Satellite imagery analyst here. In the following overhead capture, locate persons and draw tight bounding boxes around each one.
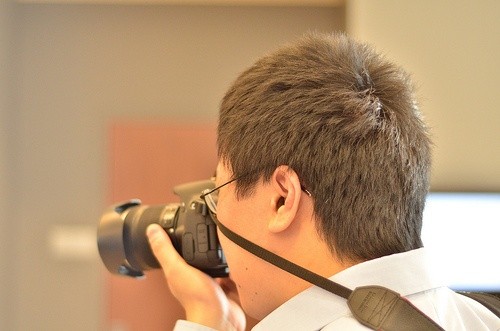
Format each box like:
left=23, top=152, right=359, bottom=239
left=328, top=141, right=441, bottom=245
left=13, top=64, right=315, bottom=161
left=146, top=31, right=500, bottom=330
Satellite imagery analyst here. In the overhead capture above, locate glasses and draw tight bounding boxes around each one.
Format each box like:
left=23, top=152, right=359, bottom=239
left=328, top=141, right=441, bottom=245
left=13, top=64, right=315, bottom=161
left=200, top=164, right=312, bottom=217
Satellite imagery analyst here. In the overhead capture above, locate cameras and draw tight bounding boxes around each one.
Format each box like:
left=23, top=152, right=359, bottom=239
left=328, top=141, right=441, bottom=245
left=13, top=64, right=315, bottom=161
left=96, top=179, right=230, bottom=279
